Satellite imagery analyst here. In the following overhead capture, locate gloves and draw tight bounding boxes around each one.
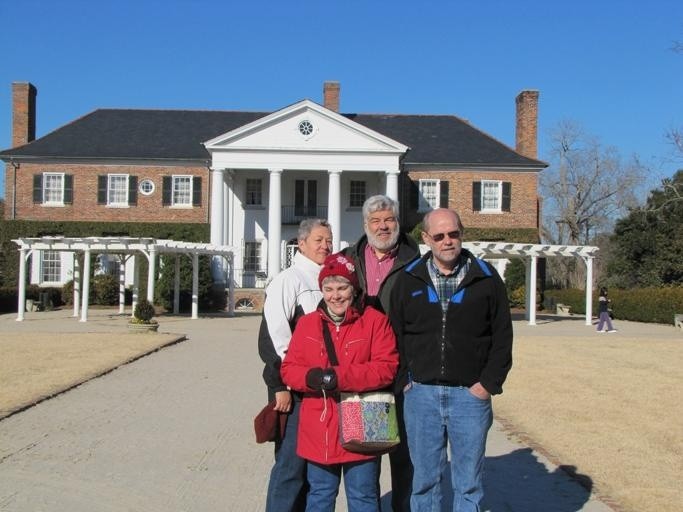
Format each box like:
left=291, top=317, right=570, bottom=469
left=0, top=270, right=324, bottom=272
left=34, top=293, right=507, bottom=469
left=306, top=368, right=337, bottom=390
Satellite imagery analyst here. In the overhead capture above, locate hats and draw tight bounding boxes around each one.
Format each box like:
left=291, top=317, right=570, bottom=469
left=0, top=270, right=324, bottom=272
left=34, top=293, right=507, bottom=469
left=318, top=253, right=360, bottom=292
left=254, top=400, right=288, bottom=443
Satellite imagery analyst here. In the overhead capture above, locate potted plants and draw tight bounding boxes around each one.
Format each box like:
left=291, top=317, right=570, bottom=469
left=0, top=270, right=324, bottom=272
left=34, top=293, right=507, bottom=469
left=128, top=299, right=158, bottom=333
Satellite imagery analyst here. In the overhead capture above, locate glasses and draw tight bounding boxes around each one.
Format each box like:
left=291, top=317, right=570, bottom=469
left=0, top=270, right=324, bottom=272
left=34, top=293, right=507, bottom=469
left=427, top=231, right=460, bottom=241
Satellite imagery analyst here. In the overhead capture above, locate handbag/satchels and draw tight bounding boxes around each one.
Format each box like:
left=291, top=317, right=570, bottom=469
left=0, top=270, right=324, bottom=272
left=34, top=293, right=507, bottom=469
left=338, top=391, right=401, bottom=455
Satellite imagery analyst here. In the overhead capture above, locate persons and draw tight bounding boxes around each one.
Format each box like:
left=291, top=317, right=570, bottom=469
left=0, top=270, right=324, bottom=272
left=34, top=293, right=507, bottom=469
left=256, top=216, right=338, bottom=511
left=279, top=251, right=399, bottom=512
left=595, top=287, right=618, bottom=332
left=385, top=206, right=513, bottom=512
left=329, top=193, right=423, bottom=512
